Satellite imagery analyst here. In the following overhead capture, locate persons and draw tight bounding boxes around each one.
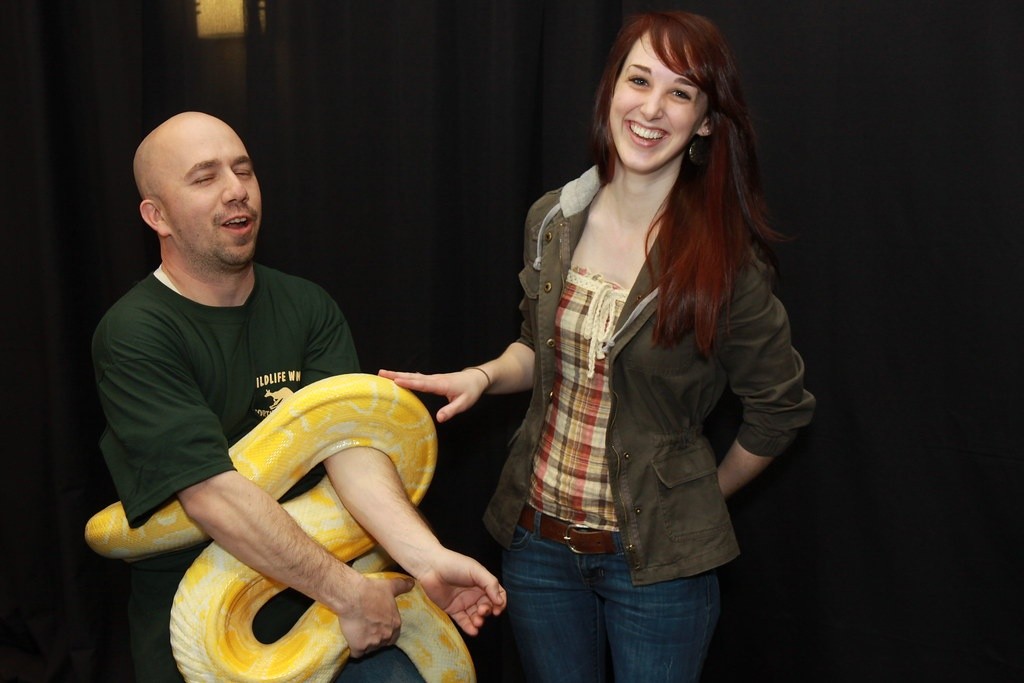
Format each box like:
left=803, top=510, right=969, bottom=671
left=377, top=11, right=816, bottom=683
left=91, top=111, right=507, bottom=683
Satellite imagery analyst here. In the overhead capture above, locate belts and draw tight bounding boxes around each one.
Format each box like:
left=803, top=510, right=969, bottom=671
left=517, top=505, right=617, bottom=554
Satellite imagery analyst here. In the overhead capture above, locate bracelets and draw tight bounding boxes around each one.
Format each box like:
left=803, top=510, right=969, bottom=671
left=464, top=367, right=491, bottom=385
